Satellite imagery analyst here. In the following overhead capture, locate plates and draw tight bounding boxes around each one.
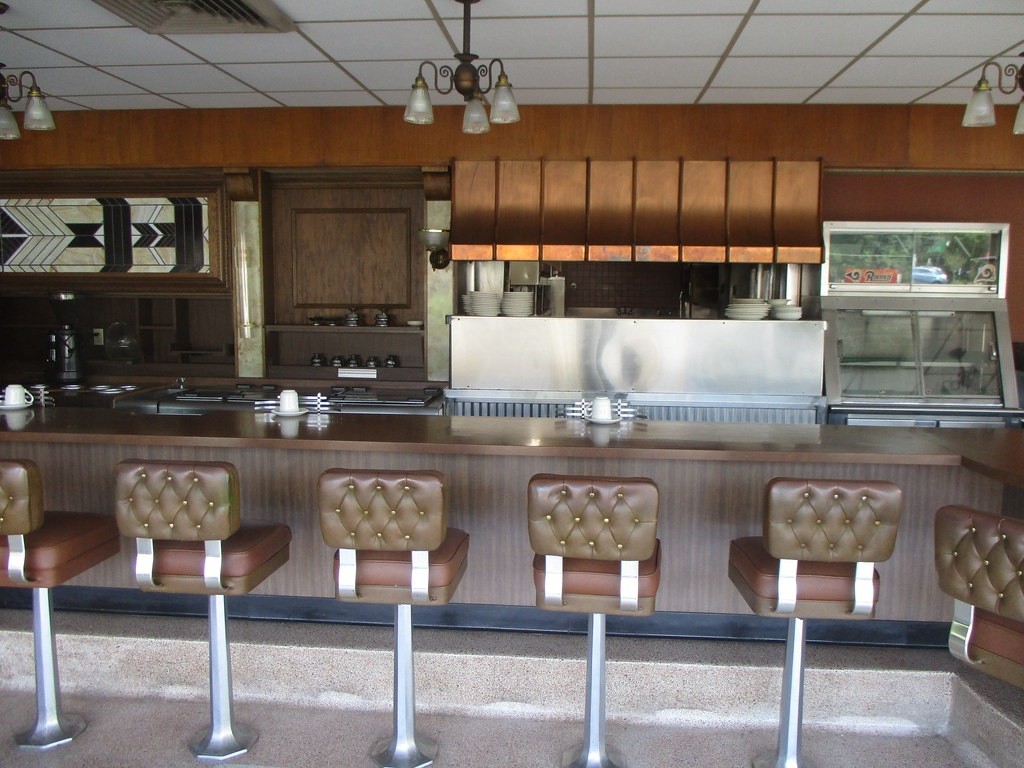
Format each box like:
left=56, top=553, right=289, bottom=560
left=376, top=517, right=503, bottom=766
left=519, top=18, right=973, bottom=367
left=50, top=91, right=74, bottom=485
left=502, top=292, right=534, bottom=316
left=271, top=408, right=308, bottom=417
left=725, top=298, right=771, bottom=320
left=586, top=416, right=622, bottom=424
left=0, top=401, right=33, bottom=410
left=407, top=321, right=423, bottom=326
left=462, top=291, right=501, bottom=316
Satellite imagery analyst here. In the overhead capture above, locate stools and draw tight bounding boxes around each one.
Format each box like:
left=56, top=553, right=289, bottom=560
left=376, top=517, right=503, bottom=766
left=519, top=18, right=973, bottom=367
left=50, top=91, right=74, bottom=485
left=112, top=457, right=292, bottom=762
left=728, top=477, right=905, bottom=768
left=0, top=457, right=122, bottom=751
left=314, top=469, right=470, bottom=768
left=528, top=472, right=660, bottom=768
left=934, top=500, right=1024, bottom=690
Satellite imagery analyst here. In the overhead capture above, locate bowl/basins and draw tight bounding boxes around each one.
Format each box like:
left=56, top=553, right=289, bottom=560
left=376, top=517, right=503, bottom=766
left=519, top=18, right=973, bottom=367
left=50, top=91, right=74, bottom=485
left=763, top=299, right=790, bottom=306
left=770, top=307, right=802, bottom=320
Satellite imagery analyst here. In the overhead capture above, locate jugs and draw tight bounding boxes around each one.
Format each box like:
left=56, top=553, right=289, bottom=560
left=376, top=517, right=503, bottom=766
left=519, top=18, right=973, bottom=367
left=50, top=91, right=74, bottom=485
left=58, top=324, right=82, bottom=384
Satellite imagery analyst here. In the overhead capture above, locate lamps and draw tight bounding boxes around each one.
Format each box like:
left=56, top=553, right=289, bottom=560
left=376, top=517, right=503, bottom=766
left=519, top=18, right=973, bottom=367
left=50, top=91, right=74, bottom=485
left=418, top=227, right=451, bottom=272
left=403, top=1, right=522, bottom=133
left=0, top=3, right=56, bottom=141
left=961, top=52, right=1024, bottom=142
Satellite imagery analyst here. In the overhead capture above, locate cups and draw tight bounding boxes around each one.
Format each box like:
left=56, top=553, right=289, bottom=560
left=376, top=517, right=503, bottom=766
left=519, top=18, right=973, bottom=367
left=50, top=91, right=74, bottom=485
left=280, top=390, right=298, bottom=412
left=5, top=385, right=34, bottom=405
left=586, top=397, right=612, bottom=420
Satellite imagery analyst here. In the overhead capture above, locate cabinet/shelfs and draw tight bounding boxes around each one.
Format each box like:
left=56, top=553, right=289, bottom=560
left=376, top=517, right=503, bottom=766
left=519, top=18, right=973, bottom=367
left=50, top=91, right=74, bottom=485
left=264, top=323, right=424, bottom=382
left=0, top=296, right=235, bottom=366
